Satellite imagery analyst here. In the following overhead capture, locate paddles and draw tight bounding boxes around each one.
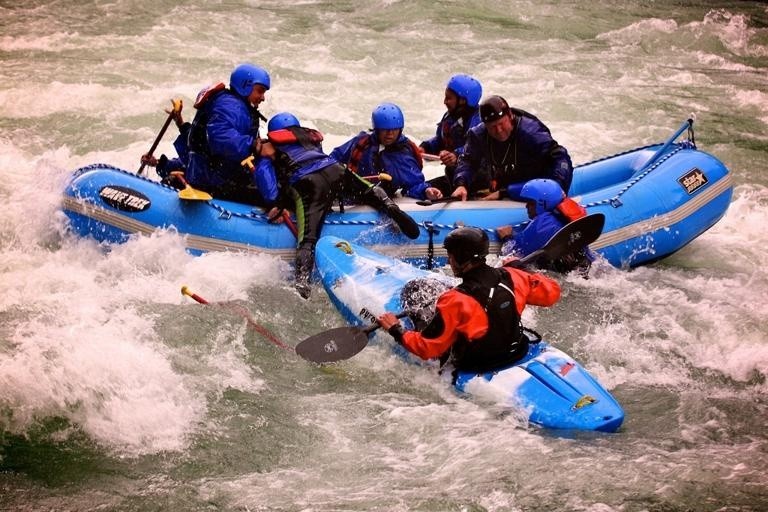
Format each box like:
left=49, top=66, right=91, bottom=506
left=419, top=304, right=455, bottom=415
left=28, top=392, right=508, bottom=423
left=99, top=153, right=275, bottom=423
left=171, top=170, right=212, bottom=200
left=296, top=213, right=608, bottom=366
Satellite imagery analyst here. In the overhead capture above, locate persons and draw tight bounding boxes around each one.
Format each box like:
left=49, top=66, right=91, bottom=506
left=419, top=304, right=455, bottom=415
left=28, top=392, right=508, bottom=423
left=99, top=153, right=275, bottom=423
left=418, top=74, right=482, bottom=198
left=141, top=99, right=191, bottom=191
left=183, top=63, right=270, bottom=205
left=449, top=95, right=574, bottom=198
left=482, top=179, right=596, bottom=280
left=376, top=227, right=560, bottom=372
left=328, top=103, right=443, bottom=201
left=267, top=112, right=420, bottom=300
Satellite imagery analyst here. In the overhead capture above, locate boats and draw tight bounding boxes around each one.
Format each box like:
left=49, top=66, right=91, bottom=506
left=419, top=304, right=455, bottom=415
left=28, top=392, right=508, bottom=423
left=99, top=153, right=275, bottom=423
left=314, top=235, right=625, bottom=434
left=62, top=117, right=734, bottom=275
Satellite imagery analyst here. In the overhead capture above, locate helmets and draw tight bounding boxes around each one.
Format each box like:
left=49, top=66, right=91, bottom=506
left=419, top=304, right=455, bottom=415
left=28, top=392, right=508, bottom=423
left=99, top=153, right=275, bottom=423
left=442, top=226, right=490, bottom=261
left=480, top=95, right=511, bottom=123
left=371, top=102, right=404, bottom=129
left=520, top=179, right=564, bottom=215
left=230, top=65, right=270, bottom=97
left=269, top=112, right=300, bottom=132
left=448, top=75, right=483, bottom=108
left=194, top=81, right=225, bottom=108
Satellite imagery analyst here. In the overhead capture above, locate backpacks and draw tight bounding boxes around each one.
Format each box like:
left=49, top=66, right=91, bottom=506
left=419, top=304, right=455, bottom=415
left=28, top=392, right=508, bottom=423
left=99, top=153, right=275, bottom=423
left=455, top=267, right=530, bottom=370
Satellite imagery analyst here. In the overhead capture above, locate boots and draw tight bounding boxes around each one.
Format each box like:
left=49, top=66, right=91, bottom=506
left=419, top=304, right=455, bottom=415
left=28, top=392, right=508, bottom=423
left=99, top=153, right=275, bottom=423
left=293, top=240, right=318, bottom=298
left=368, top=186, right=421, bottom=239
